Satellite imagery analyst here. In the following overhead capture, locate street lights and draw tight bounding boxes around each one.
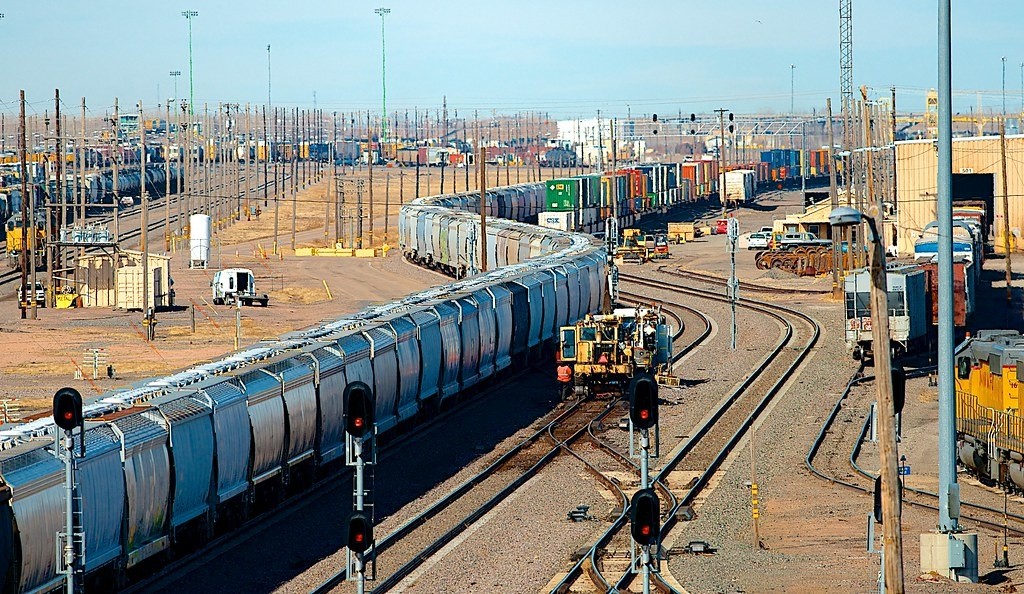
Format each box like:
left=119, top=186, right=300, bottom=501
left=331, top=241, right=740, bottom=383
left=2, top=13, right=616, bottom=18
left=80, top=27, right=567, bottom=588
left=170, top=70, right=181, bottom=137
left=1019, top=62, right=1024, bottom=112
left=181, top=9, right=199, bottom=120
left=829, top=206, right=905, bottom=593
left=1001, top=56, right=1008, bottom=115
left=375, top=7, right=392, bottom=141
left=788, top=63, right=797, bottom=113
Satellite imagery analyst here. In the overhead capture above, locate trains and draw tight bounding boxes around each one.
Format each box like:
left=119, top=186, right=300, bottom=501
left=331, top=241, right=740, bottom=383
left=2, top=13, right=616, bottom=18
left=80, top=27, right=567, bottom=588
left=841, top=194, right=990, bottom=363
left=1, top=146, right=612, bottom=593
left=953, top=329, right=1024, bottom=498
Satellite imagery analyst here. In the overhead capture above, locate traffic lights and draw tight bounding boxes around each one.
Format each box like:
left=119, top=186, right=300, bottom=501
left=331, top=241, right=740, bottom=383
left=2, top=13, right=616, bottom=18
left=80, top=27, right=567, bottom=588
left=627, top=372, right=659, bottom=429
left=343, top=381, right=374, bottom=438
left=630, top=488, right=661, bottom=545
left=53, top=386, right=83, bottom=430
left=346, top=510, right=373, bottom=553
